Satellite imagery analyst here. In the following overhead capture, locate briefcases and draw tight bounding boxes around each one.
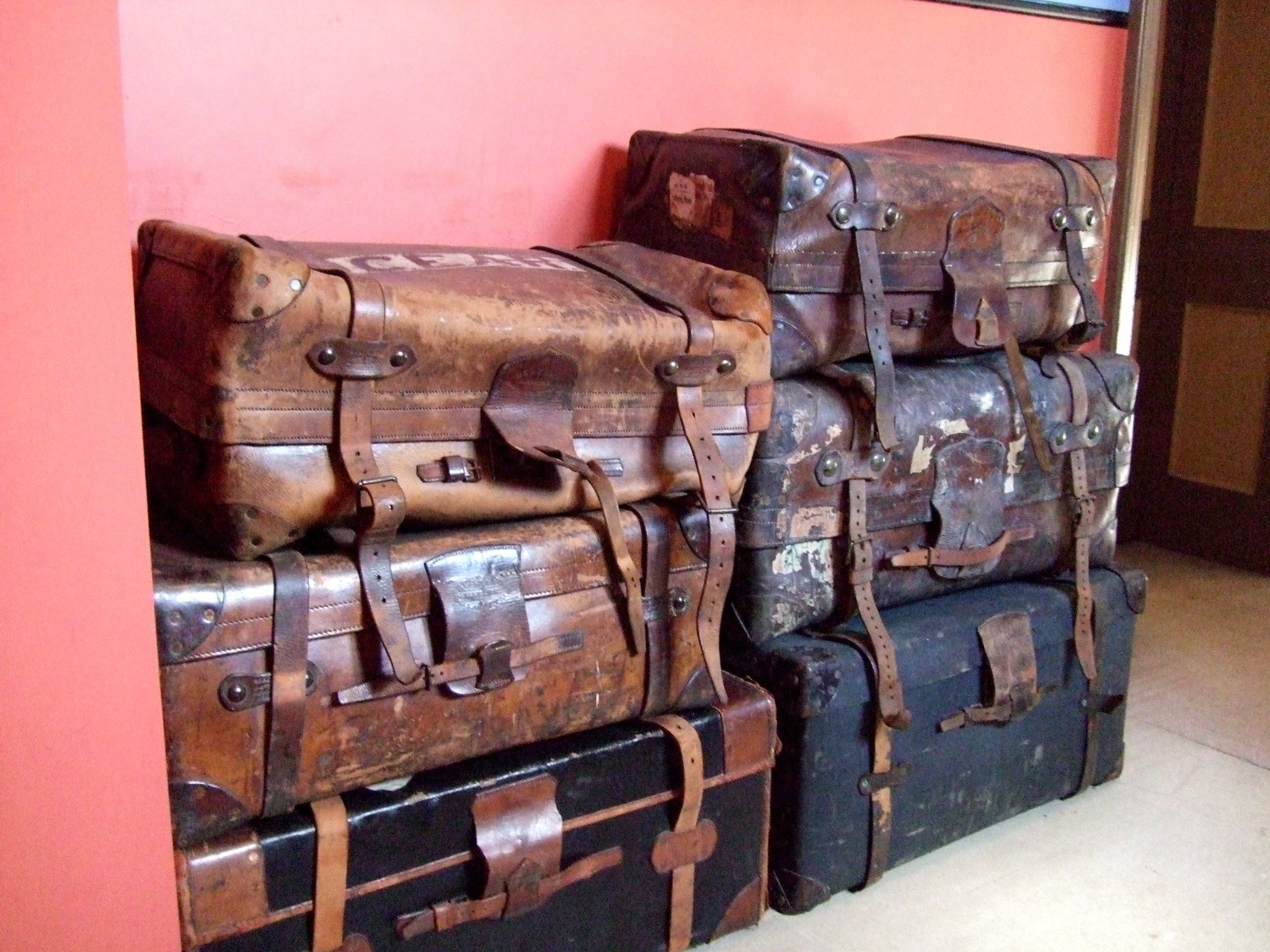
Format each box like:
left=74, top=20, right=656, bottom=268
left=128, top=126, right=1147, bottom=946
